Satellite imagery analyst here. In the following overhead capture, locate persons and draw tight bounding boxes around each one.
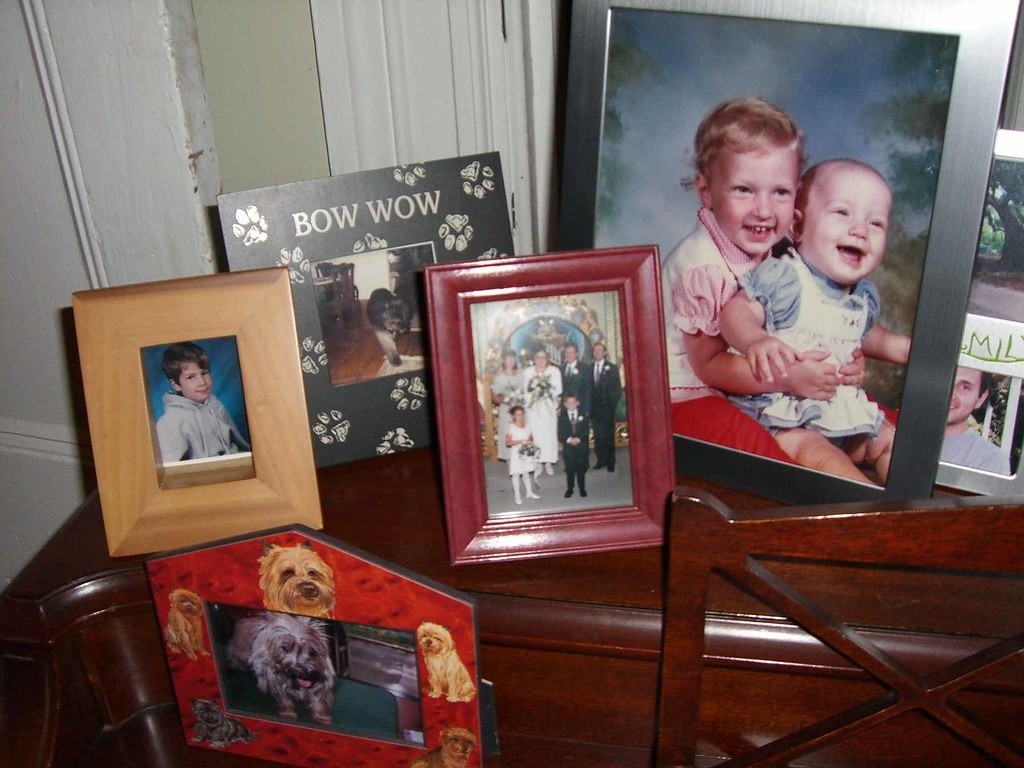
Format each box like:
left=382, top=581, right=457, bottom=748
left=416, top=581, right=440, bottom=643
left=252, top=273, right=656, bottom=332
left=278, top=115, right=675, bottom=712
left=152, top=94, right=1012, bottom=505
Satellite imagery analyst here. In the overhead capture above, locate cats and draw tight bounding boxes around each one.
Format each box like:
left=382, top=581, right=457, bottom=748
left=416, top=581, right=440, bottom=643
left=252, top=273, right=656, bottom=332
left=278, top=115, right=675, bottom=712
left=366, top=288, right=413, bottom=368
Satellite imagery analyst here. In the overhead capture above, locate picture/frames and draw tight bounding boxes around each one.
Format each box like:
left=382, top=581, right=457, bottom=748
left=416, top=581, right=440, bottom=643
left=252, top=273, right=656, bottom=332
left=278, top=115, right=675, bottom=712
left=935, top=364, right=1024, bottom=494
left=958, top=128, right=1024, bottom=378
left=143, top=524, right=483, bottom=768
left=70, top=265, right=323, bottom=557
left=425, top=244, right=680, bottom=566
left=555, top=0, right=1021, bottom=507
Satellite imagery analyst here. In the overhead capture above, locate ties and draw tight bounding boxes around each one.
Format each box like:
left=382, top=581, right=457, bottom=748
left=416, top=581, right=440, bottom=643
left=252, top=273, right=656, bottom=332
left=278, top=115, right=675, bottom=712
left=572, top=414, right=574, bottom=421
left=597, top=363, right=599, bottom=374
left=568, top=367, right=570, bottom=375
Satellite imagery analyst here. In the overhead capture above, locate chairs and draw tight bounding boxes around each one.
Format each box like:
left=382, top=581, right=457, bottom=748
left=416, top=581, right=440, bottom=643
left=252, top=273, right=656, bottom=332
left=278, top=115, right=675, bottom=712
left=654, top=485, right=1024, bottom=768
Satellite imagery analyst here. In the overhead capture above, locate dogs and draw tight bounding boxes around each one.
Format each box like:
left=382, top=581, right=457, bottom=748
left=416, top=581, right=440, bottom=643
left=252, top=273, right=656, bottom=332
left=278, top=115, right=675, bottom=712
left=228, top=610, right=337, bottom=725
left=189, top=697, right=263, bottom=749
left=417, top=619, right=476, bottom=703
left=408, top=727, right=477, bottom=768
left=256, top=542, right=337, bottom=619
left=164, top=589, right=210, bottom=661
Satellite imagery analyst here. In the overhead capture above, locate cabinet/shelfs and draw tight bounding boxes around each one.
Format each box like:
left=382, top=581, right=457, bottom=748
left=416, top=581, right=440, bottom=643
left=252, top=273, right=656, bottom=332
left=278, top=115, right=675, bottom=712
left=0, top=446, right=1024, bottom=768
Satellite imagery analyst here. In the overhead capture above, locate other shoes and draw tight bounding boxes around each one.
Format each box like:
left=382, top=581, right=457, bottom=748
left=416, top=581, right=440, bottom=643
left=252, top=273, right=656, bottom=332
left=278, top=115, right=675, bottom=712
left=537, top=465, right=543, bottom=475
left=593, top=462, right=607, bottom=470
left=545, top=465, right=554, bottom=475
left=565, top=489, right=572, bottom=497
left=581, top=489, right=587, bottom=497
left=514, top=497, right=522, bottom=504
left=608, top=464, right=614, bottom=472
left=526, top=493, right=539, bottom=499
